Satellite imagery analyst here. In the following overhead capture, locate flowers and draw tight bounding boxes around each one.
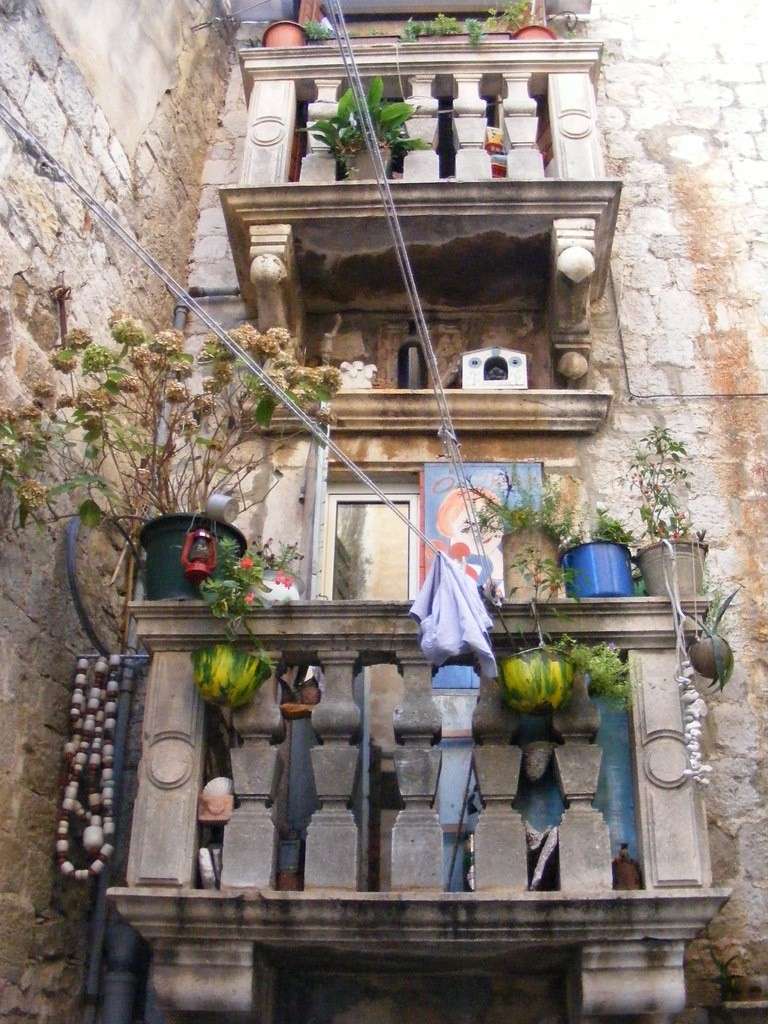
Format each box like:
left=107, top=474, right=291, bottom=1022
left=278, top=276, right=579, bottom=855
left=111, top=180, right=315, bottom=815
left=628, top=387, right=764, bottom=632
left=614, top=423, right=700, bottom=544
left=506, top=544, right=638, bottom=711
left=0, top=307, right=346, bottom=527
left=247, top=534, right=306, bottom=574
left=199, top=532, right=305, bottom=667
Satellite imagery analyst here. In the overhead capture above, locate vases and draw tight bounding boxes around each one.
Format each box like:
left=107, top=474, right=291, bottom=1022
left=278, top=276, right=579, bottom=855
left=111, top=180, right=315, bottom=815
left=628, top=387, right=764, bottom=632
left=139, top=513, right=247, bottom=602
left=493, top=646, right=580, bottom=715
left=635, top=538, right=709, bottom=596
left=247, top=569, right=306, bottom=604
left=190, top=642, right=273, bottom=709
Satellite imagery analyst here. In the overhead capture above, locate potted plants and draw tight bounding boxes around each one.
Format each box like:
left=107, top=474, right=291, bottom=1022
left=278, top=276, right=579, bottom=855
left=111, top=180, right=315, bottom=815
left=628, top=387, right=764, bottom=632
left=501, top=0, right=557, bottom=42
left=294, top=76, right=431, bottom=181
left=262, top=19, right=335, bottom=47
left=680, top=582, right=740, bottom=693
left=460, top=453, right=593, bottom=602
left=397, top=13, right=512, bottom=46
left=552, top=506, right=637, bottom=599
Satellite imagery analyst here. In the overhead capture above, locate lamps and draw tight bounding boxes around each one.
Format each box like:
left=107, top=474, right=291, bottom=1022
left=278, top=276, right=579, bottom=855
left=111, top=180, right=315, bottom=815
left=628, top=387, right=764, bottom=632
left=181, top=528, right=218, bottom=581
